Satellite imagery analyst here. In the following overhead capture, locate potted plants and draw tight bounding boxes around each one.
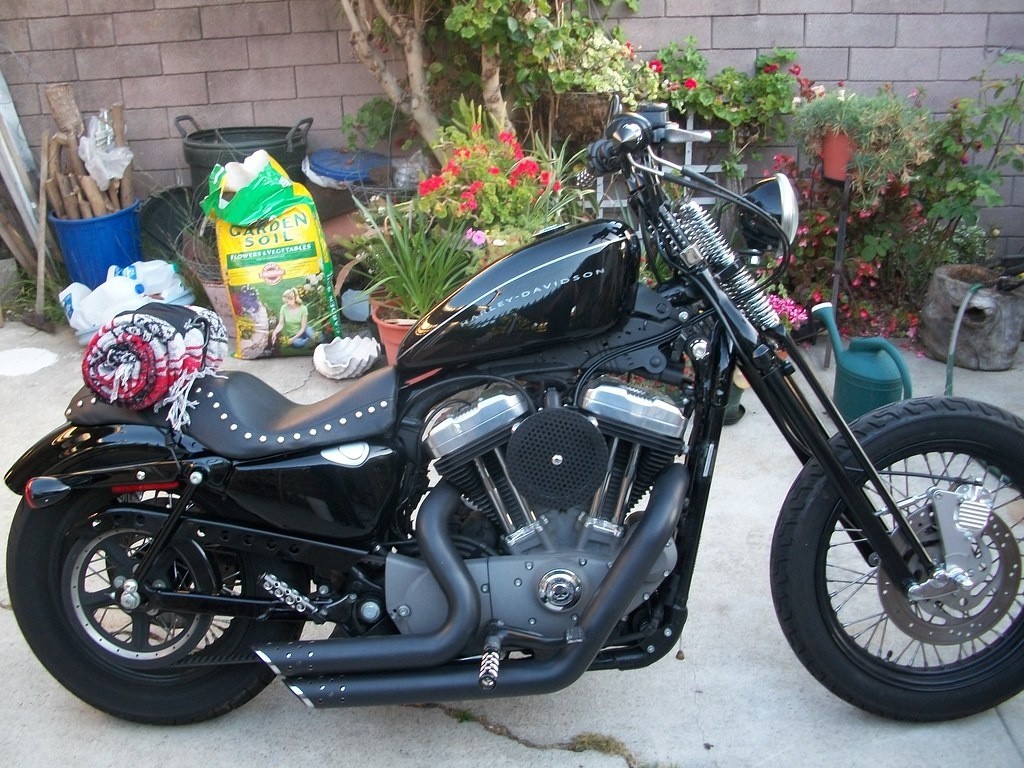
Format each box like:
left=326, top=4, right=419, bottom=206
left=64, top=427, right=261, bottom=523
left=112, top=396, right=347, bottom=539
left=347, top=194, right=482, bottom=367
left=797, top=88, right=941, bottom=215
left=423, top=0, right=640, bottom=143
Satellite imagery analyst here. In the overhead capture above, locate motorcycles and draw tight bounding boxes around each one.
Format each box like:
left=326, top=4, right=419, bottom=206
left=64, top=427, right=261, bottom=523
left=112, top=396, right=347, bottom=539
left=3, top=95, right=1024, bottom=726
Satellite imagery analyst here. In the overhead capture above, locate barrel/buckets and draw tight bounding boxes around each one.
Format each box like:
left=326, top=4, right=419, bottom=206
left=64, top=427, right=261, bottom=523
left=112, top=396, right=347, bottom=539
left=172, top=112, right=394, bottom=245
left=48, top=196, right=147, bottom=295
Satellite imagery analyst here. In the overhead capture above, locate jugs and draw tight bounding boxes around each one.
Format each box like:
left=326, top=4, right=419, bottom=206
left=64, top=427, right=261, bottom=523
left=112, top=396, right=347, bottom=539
left=810, top=299, right=914, bottom=429
